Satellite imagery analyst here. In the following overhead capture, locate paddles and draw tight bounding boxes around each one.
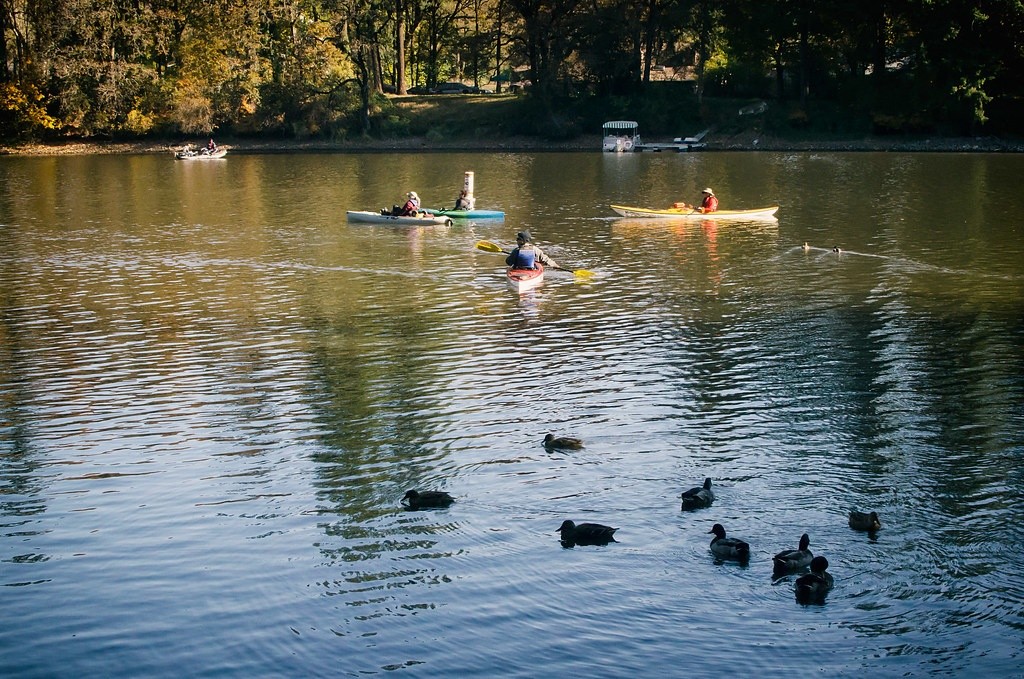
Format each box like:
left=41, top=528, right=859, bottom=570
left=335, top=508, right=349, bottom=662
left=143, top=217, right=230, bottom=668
left=476, top=239, right=594, bottom=274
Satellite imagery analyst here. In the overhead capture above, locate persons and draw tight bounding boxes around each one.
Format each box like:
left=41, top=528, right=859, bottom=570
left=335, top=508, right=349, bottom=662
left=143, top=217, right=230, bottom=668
left=393, top=191, right=421, bottom=216
left=184, top=138, right=217, bottom=157
left=696, top=187, right=718, bottom=213
left=505, top=233, right=561, bottom=271
left=452, top=190, right=471, bottom=210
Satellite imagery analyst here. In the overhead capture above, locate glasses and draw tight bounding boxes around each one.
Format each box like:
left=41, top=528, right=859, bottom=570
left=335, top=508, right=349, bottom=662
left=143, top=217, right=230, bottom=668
left=516, top=237, right=522, bottom=242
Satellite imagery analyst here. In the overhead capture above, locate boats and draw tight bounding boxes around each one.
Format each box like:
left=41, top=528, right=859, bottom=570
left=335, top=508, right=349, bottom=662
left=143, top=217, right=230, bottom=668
left=345, top=210, right=456, bottom=227
left=418, top=206, right=506, bottom=218
left=602, top=121, right=641, bottom=152
left=609, top=204, right=779, bottom=218
left=173, top=145, right=228, bottom=159
left=506, top=262, right=544, bottom=292
left=673, top=129, right=710, bottom=144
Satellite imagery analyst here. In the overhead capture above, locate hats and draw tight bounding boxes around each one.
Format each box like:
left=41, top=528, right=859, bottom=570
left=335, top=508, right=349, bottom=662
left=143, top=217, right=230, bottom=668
left=407, top=190, right=417, bottom=197
left=702, top=187, right=715, bottom=196
left=517, top=231, right=532, bottom=242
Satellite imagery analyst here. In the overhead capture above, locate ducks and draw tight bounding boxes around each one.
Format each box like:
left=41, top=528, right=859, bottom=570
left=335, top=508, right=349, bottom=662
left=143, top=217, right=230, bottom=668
left=795, top=556, right=834, bottom=591
left=708, top=523, right=750, bottom=559
left=682, top=477, right=713, bottom=504
left=555, top=520, right=620, bottom=539
left=542, top=434, right=582, bottom=449
left=833, top=245, right=842, bottom=252
left=849, top=509, right=880, bottom=530
left=802, top=242, right=810, bottom=250
left=401, top=490, right=455, bottom=507
left=772, top=533, right=813, bottom=568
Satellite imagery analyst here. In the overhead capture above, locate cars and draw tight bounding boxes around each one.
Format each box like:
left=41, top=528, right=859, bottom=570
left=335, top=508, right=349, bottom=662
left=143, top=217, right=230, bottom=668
left=429, top=82, right=473, bottom=94
left=738, top=98, right=769, bottom=115
left=406, top=86, right=432, bottom=95
